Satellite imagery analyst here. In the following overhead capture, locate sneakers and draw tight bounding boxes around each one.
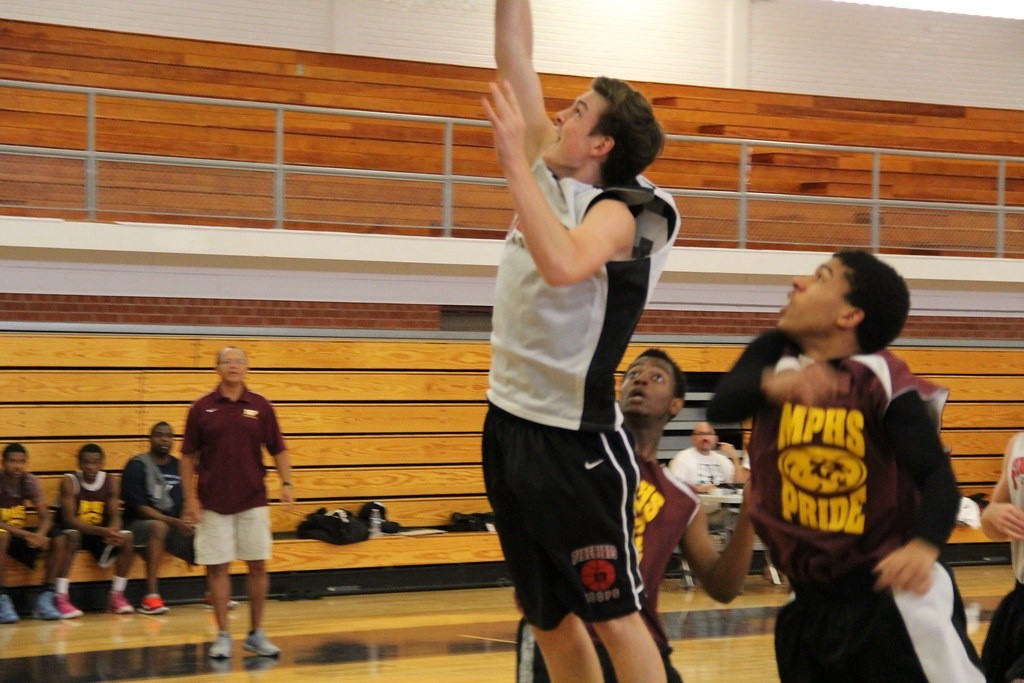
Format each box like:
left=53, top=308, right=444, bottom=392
left=105, top=592, right=134, bottom=614
left=30, top=591, right=62, bottom=619
left=208, top=631, right=234, bottom=660
left=135, top=594, right=170, bottom=616
left=55, top=594, right=83, bottom=618
left=206, top=593, right=239, bottom=608
left=0, top=595, right=19, bottom=623
left=243, top=628, right=280, bottom=658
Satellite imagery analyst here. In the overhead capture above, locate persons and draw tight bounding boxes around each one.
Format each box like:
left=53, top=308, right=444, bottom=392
left=54, top=444, right=134, bottom=618
left=120, top=421, right=239, bottom=613
left=482, top=0, right=681, bottom=683
left=0, top=443, right=59, bottom=623
left=980, top=431, right=1024, bottom=683
left=706, top=252, right=995, bottom=683
left=516, top=349, right=756, bottom=683
left=180, top=345, right=292, bottom=657
left=669, top=421, right=741, bottom=494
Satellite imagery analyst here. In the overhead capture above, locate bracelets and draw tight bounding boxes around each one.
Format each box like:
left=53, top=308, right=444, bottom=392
left=283, top=481, right=290, bottom=486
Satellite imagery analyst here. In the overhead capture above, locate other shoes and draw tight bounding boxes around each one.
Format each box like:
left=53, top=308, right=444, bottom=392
left=760, top=567, right=781, bottom=585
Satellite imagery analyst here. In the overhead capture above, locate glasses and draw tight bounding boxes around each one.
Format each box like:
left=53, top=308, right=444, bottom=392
left=220, top=357, right=248, bottom=367
left=689, top=429, right=719, bottom=437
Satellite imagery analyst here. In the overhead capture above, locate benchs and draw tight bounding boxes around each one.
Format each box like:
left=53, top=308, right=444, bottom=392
left=0, top=333, right=1024, bottom=589
